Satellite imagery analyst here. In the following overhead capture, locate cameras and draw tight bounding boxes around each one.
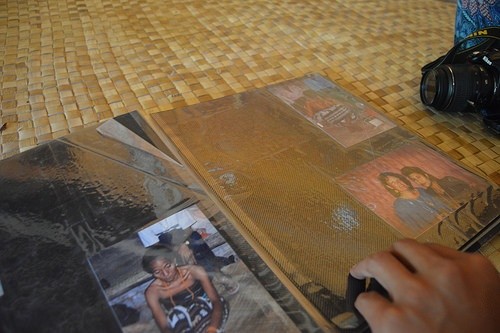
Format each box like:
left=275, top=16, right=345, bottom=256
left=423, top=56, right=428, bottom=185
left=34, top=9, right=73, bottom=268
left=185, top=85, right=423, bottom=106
left=419, top=43, right=500, bottom=137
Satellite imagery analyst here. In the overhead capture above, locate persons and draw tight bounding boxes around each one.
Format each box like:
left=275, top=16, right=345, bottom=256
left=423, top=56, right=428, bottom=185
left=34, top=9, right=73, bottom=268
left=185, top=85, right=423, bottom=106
left=378, top=171, right=456, bottom=235
left=141, top=244, right=230, bottom=332
left=401, top=166, right=477, bottom=210
left=303, top=87, right=375, bottom=137
left=349, top=237, right=500, bottom=332
